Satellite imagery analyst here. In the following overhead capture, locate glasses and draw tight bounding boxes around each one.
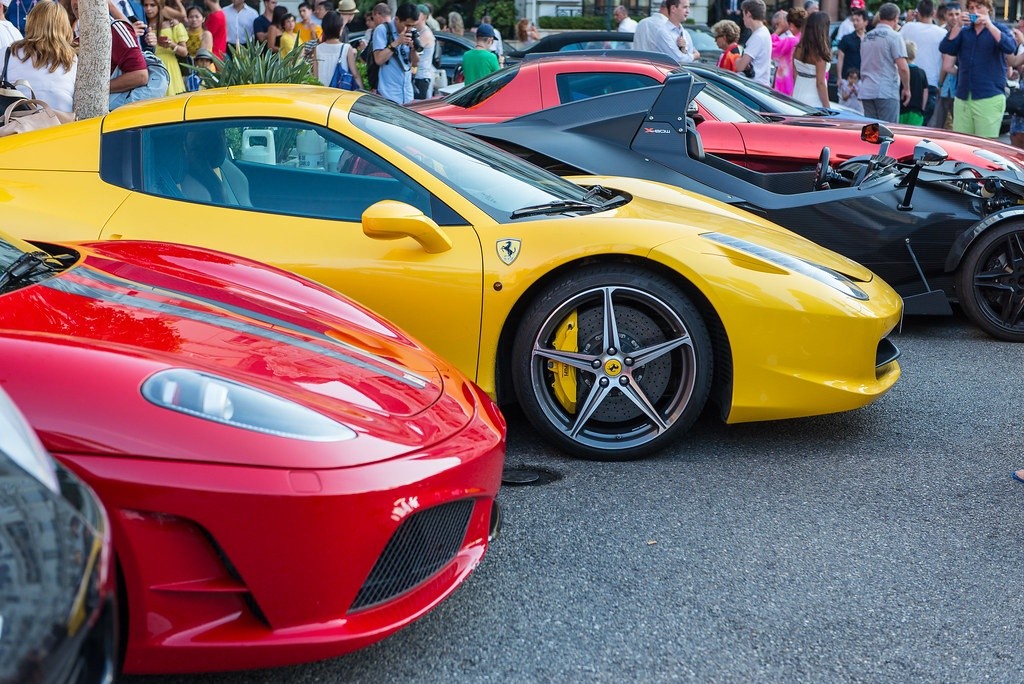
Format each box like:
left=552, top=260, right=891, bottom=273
left=714, top=35, right=724, bottom=41
left=944, top=4, right=961, bottom=10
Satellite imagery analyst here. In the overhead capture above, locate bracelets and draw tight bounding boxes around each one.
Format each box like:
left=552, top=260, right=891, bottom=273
left=170, top=44, right=178, bottom=53
left=499, top=62, right=504, bottom=65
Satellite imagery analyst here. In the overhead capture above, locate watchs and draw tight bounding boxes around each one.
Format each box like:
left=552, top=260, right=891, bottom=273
left=386, top=43, right=397, bottom=53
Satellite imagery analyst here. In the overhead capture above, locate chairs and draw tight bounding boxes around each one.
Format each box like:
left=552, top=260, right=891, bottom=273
left=154, top=128, right=212, bottom=202
left=188, top=125, right=253, bottom=207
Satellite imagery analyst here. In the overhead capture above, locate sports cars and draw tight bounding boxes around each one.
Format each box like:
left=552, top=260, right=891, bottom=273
left=324, top=25, right=1024, bottom=347
left=1, top=79, right=909, bottom=468
left=0, top=232, right=508, bottom=684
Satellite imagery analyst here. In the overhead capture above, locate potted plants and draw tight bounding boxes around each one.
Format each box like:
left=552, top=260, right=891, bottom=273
left=179, top=25, right=325, bottom=170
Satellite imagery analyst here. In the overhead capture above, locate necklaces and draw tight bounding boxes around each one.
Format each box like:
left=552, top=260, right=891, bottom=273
left=474, top=44, right=486, bottom=50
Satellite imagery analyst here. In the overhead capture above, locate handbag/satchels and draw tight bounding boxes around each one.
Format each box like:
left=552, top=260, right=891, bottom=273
left=0, top=75, right=76, bottom=138
left=361, top=21, right=393, bottom=89
left=107, top=18, right=170, bottom=113
left=1005, top=90, right=1024, bottom=116
left=330, top=43, right=359, bottom=90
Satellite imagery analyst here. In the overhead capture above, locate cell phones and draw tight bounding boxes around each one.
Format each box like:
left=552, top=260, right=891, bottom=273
left=967, top=14, right=977, bottom=23
left=128, top=16, right=137, bottom=23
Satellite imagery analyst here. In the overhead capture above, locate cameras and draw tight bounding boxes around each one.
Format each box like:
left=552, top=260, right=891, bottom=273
left=406, top=28, right=424, bottom=53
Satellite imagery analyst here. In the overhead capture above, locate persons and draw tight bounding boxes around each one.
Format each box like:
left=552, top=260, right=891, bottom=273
left=0, top=0, right=544, bottom=121
left=938, top=0, right=1016, bottom=138
left=462, top=24, right=505, bottom=88
left=372, top=3, right=420, bottom=105
left=790, top=12, right=835, bottom=109
left=606, top=0, right=1024, bottom=152
left=858, top=2, right=911, bottom=120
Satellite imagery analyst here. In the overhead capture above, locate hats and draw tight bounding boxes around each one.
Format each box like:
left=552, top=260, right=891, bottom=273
left=336, top=0, right=359, bottom=14
left=477, top=24, right=498, bottom=41
left=417, top=3, right=430, bottom=19
left=194, top=48, right=214, bottom=62
left=850, top=0, right=866, bottom=9
